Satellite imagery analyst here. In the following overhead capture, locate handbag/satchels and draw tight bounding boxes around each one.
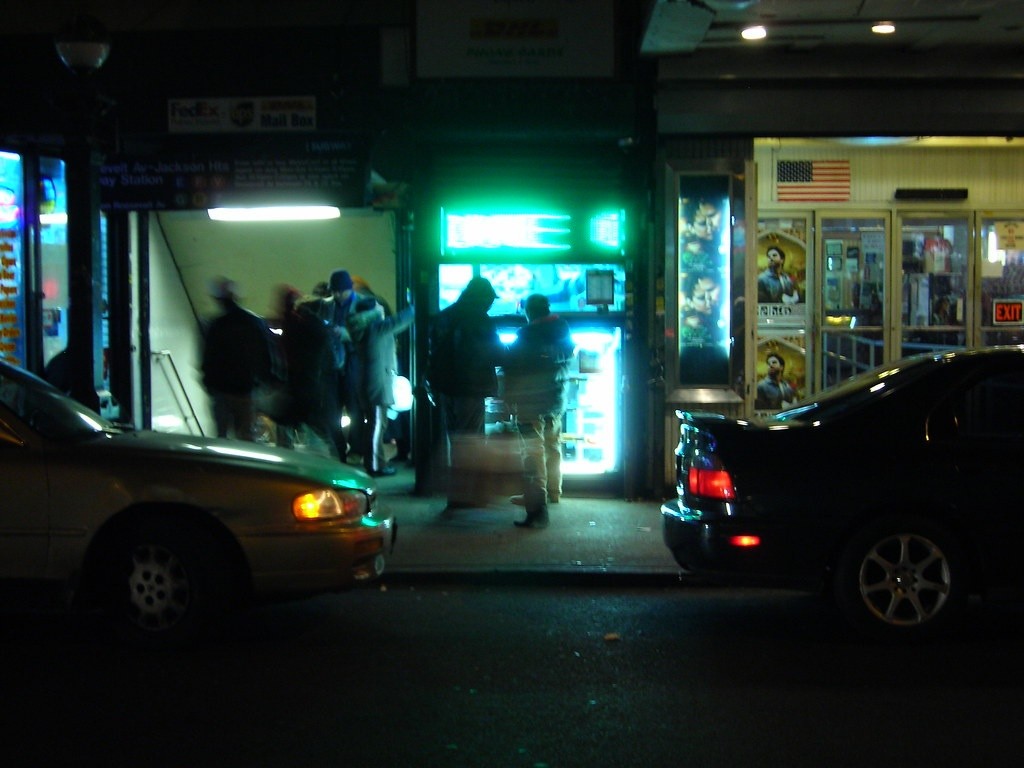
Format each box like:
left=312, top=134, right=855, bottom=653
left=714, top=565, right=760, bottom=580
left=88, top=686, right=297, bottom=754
left=484, top=410, right=527, bottom=496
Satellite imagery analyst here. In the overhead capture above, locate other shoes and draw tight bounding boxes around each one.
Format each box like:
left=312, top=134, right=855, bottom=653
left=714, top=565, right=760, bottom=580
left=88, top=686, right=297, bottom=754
left=547, top=493, right=559, bottom=503
left=373, top=465, right=398, bottom=477
left=513, top=517, right=545, bottom=529
left=509, top=492, right=524, bottom=506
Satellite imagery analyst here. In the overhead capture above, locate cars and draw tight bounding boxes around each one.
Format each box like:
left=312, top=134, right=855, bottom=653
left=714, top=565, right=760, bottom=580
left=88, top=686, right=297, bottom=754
left=0, top=359, right=397, bottom=640
left=659, top=345, right=1023, bottom=645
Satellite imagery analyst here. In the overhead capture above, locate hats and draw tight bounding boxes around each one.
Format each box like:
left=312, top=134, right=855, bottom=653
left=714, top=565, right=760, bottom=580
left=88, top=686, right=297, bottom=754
left=467, top=277, right=499, bottom=298
left=326, top=271, right=353, bottom=294
left=206, top=275, right=235, bottom=299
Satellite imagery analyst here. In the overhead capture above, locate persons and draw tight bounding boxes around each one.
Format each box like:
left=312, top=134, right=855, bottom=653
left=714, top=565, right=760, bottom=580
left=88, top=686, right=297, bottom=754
left=678, top=197, right=729, bottom=347
left=425, top=275, right=576, bottom=529
left=756, top=352, right=799, bottom=409
left=273, top=269, right=408, bottom=477
left=758, top=243, right=800, bottom=304
left=193, top=275, right=274, bottom=442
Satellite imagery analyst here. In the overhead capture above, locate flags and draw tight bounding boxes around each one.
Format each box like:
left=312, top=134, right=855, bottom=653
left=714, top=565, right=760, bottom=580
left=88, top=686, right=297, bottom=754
left=776, top=159, right=850, bottom=203
